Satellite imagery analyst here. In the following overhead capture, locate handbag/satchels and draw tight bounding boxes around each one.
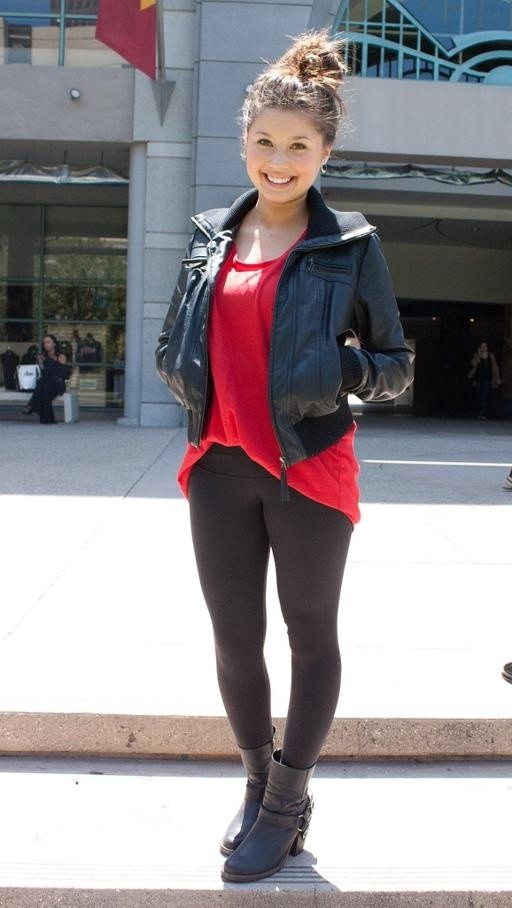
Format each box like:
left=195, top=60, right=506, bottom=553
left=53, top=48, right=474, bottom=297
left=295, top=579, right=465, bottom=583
left=13, top=364, right=39, bottom=390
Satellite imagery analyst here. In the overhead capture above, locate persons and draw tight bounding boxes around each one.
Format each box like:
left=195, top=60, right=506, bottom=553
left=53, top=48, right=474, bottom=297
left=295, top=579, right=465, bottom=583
left=151, top=36, right=418, bottom=885
left=465, top=341, right=502, bottom=423
left=20, top=332, right=73, bottom=425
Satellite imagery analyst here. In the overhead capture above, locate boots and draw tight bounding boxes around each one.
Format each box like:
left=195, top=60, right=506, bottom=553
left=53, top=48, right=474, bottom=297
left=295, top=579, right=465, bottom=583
left=219, top=724, right=282, bottom=853
left=222, top=748, right=313, bottom=881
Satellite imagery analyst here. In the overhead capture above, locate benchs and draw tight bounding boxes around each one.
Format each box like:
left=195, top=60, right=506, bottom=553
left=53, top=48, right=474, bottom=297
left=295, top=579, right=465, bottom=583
left=0, top=365, right=82, bottom=424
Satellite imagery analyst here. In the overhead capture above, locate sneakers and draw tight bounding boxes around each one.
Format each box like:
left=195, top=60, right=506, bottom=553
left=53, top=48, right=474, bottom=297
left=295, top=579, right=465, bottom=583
left=500, top=659, right=512, bottom=685
left=21, top=405, right=58, bottom=424
